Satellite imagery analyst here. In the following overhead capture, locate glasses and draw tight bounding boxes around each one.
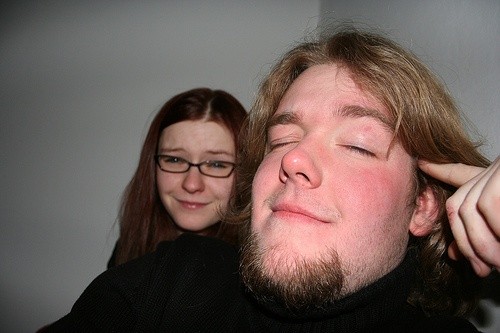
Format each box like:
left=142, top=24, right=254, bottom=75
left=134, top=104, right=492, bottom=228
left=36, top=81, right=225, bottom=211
left=153, top=154, right=237, bottom=178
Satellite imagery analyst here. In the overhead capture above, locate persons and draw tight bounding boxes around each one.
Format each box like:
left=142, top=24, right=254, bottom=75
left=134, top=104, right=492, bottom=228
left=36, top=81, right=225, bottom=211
left=107, top=87, right=247, bottom=272
left=34, top=30, right=500, bottom=333
